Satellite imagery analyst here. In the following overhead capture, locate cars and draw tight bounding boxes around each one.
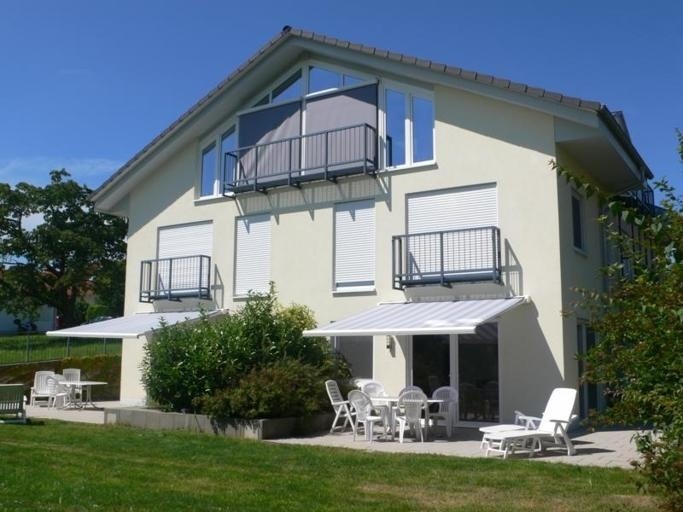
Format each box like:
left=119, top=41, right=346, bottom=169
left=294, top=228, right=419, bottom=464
left=79, top=315, right=114, bottom=326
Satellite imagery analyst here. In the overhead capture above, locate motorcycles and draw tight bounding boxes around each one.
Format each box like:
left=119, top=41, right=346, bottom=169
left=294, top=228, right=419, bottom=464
left=12, top=319, right=37, bottom=334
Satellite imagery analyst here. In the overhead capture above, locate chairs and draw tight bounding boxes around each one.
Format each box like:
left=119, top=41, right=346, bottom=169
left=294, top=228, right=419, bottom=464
left=0, top=384, right=27, bottom=425
left=324, top=380, right=458, bottom=444
left=29, top=368, right=81, bottom=411
left=477, top=387, right=578, bottom=461
left=428, top=376, right=497, bottom=420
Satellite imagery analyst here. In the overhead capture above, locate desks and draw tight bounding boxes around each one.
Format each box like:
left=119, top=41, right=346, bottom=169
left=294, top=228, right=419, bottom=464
left=58, top=381, right=107, bottom=410
